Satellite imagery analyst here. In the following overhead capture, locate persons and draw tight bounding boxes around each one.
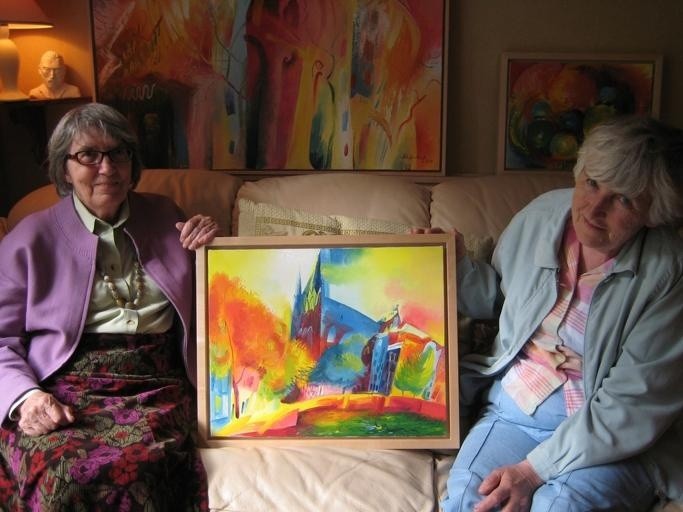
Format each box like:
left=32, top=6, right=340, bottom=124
left=1, top=101, right=219, bottom=511
left=26, top=49, right=82, bottom=99
left=405, top=118, right=679, bottom=512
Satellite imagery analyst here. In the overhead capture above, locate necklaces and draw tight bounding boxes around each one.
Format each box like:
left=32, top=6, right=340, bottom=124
left=95, top=249, right=145, bottom=311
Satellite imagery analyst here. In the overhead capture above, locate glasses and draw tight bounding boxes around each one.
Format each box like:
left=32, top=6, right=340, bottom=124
left=66, top=146, right=134, bottom=165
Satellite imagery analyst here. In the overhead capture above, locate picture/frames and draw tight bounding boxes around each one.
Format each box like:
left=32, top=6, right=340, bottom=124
left=87, top=1, right=450, bottom=178
left=195, top=233, right=461, bottom=451
left=496, top=51, right=664, bottom=172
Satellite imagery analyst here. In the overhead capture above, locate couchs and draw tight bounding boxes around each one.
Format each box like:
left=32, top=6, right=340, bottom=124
left=0, top=168, right=681, bottom=512
